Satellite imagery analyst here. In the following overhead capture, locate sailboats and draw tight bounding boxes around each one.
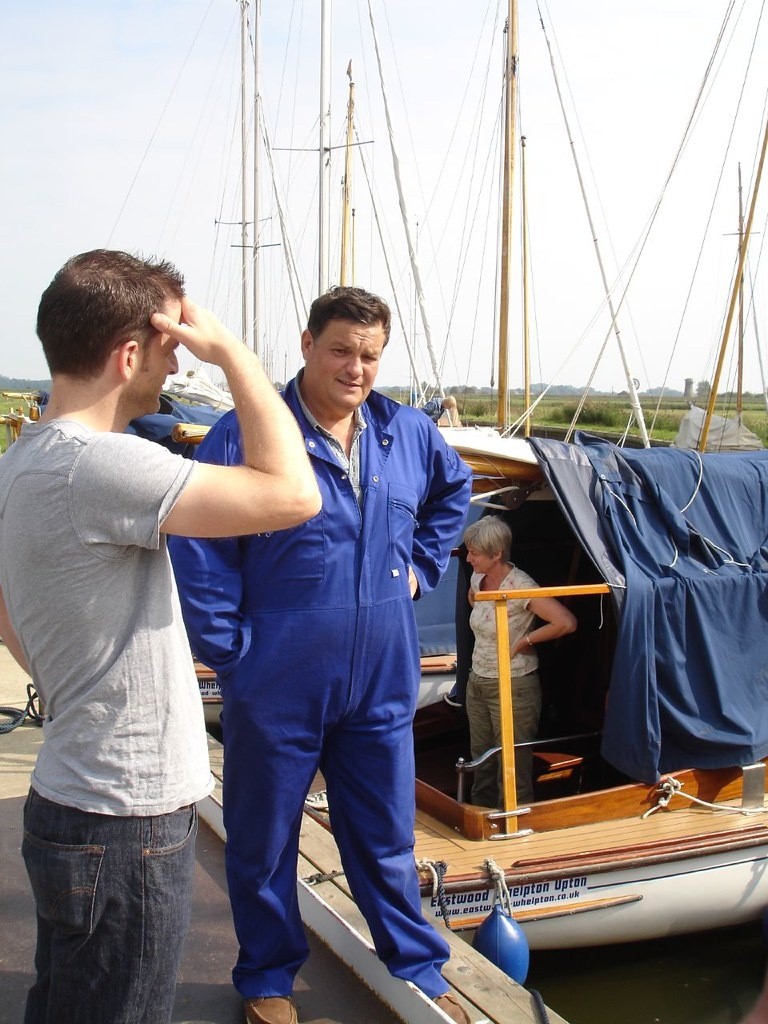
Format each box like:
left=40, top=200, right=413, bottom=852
left=0, top=0, right=768, bottom=953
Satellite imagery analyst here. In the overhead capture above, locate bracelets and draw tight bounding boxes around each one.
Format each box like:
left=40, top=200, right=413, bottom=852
left=526, top=632, right=533, bottom=645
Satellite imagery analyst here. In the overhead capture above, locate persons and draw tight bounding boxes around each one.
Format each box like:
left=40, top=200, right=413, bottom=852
left=0, top=249, right=323, bottom=1024
left=420, top=395, right=456, bottom=424
left=167, top=287, right=473, bottom=1024
left=464, top=515, right=578, bottom=808
